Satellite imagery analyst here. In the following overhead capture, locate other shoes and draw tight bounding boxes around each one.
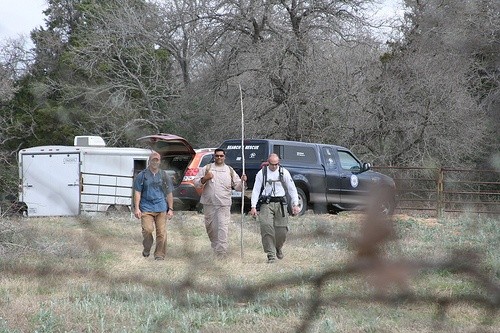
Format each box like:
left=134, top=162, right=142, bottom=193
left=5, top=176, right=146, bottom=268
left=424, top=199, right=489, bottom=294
left=268, top=259, right=275, bottom=263
left=155, top=257, right=164, bottom=261
left=143, top=251, right=150, bottom=257
left=276, top=248, right=283, bottom=259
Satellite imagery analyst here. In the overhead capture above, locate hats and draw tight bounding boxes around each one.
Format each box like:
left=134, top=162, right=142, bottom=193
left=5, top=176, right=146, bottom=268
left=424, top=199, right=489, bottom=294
left=149, top=153, right=160, bottom=160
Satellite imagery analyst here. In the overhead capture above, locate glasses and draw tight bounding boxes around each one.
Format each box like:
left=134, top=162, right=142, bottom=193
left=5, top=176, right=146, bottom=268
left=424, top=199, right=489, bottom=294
left=268, top=161, right=279, bottom=166
left=215, top=155, right=224, bottom=158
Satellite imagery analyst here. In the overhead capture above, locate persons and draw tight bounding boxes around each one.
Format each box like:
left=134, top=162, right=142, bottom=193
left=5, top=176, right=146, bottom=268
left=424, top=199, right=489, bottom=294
left=133, top=153, right=173, bottom=260
left=251, top=153, right=299, bottom=262
left=194, top=149, right=247, bottom=255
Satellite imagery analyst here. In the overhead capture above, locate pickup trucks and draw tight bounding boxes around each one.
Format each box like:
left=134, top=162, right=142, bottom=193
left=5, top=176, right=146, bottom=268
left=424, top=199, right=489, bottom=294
left=208, top=138, right=398, bottom=218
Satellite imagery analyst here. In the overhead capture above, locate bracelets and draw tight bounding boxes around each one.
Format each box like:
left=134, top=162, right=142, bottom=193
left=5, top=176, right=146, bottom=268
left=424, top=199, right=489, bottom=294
left=169, top=208, right=173, bottom=211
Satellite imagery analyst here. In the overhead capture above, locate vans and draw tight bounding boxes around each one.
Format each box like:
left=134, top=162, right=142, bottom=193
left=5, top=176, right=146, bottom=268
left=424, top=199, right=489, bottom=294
left=17, top=135, right=153, bottom=218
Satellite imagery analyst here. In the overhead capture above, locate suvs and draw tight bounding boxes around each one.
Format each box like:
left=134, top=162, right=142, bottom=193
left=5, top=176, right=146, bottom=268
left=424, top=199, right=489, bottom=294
left=135, top=132, right=218, bottom=211
